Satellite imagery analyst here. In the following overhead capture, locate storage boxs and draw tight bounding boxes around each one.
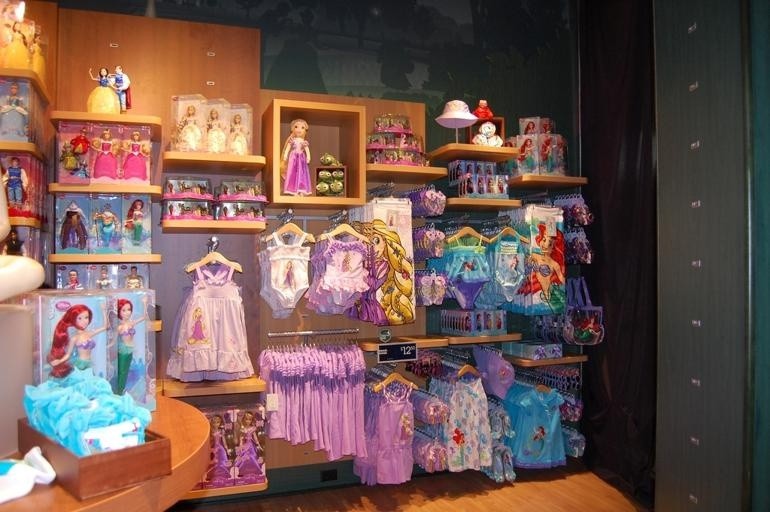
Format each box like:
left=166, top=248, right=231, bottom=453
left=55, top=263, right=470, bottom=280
left=17, top=416, right=172, bottom=503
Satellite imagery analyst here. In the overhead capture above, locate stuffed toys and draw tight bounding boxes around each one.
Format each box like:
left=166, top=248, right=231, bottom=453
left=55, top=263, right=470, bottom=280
left=367, top=114, right=433, bottom=167
left=60, top=143, right=81, bottom=174
left=315, top=153, right=348, bottom=197
left=68, top=128, right=91, bottom=154
left=68, top=161, right=90, bottom=178
left=472, top=99, right=494, bottom=119
left=472, top=123, right=504, bottom=147
left=0, top=0, right=46, bottom=79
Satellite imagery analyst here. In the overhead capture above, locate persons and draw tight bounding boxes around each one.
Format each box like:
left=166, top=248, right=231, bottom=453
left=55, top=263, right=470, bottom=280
left=441, top=309, right=507, bottom=337
left=57, top=202, right=87, bottom=249
left=120, top=131, right=152, bottom=182
left=533, top=277, right=604, bottom=345
left=280, top=119, right=312, bottom=197
left=95, top=265, right=112, bottom=292
left=203, top=413, right=233, bottom=482
left=161, top=175, right=269, bottom=222
left=1, top=226, right=47, bottom=286
left=123, top=199, right=150, bottom=247
left=123, top=265, right=148, bottom=290
left=236, top=412, right=263, bottom=477
left=174, top=101, right=203, bottom=150
left=110, top=64, right=131, bottom=114
left=505, top=118, right=568, bottom=177
left=1, top=150, right=48, bottom=224
left=227, top=115, right=251, bottom=156
left=114, top=294, right=148, bottom=396
left=203, top=107, right=226, bottom=152
left=447, top=160, right=510, bottom=199
left=50, top=306, right=108, bottom=380
left=90, top=127, right=122, bottom=183
left=64, top=269, right=81, bottom=292
left=87, top=64, right=119, bottom=114
left=91, top=203, right=121, bottom=246
left=0, top=79, right=44, bottom=148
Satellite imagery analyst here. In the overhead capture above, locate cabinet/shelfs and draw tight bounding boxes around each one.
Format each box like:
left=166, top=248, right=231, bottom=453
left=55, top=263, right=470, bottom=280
left=0, top=2, right=590, bottom=512
